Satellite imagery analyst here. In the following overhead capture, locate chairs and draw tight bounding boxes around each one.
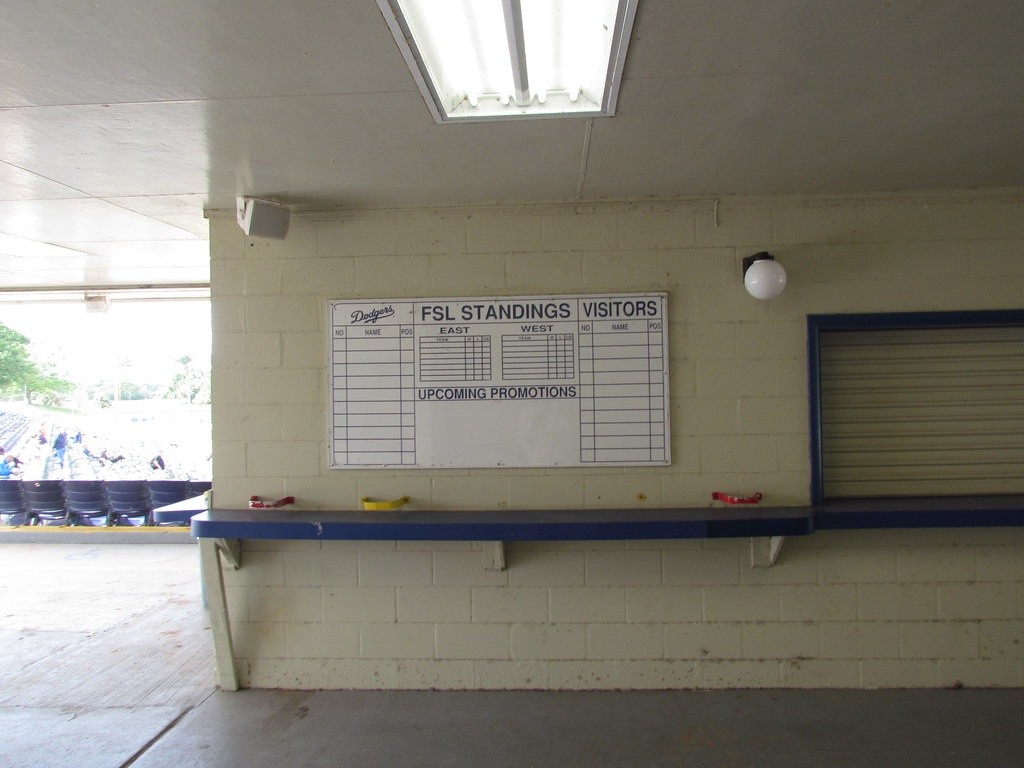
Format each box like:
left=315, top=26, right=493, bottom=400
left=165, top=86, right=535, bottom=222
left=0, top=408, right=213, bottom=526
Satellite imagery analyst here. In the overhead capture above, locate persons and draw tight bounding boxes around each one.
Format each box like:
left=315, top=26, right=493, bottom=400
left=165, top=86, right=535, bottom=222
left=0, top=420, right=212, bottom=480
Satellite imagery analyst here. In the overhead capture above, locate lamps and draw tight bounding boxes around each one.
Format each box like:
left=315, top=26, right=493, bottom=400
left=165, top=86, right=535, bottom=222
left=236, top=197, right=292, bottom=243
left=741, top=251, right=789, bottom=302
